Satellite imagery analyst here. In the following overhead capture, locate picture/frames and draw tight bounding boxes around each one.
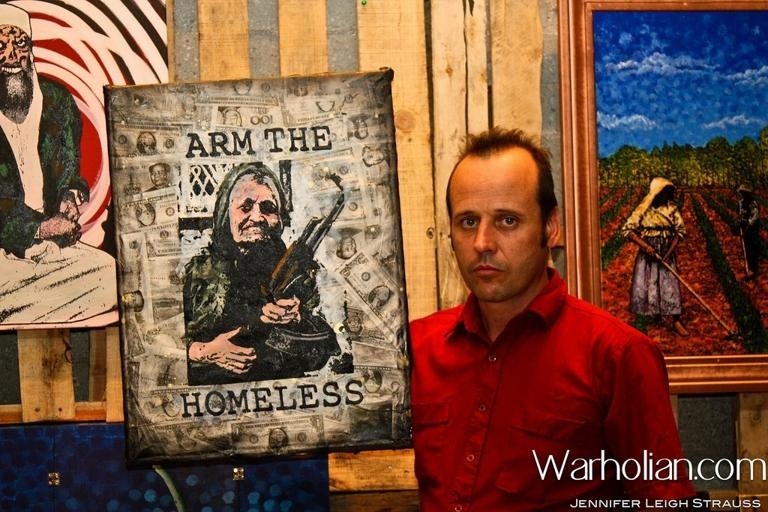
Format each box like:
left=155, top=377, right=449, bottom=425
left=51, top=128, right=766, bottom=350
left=557, top=0, right=767, bottom=396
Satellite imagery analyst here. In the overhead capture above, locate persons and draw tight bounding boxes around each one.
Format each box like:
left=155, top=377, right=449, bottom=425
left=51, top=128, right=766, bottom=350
left=733, top=180, right=762, bottom=280
left=405, top=121, right=697, bottom=511
left=1, top=2, right=118, bottom=321
left=621, top=167, right=694, bottom=338
left=103, top=73, right=410, bottom=454
